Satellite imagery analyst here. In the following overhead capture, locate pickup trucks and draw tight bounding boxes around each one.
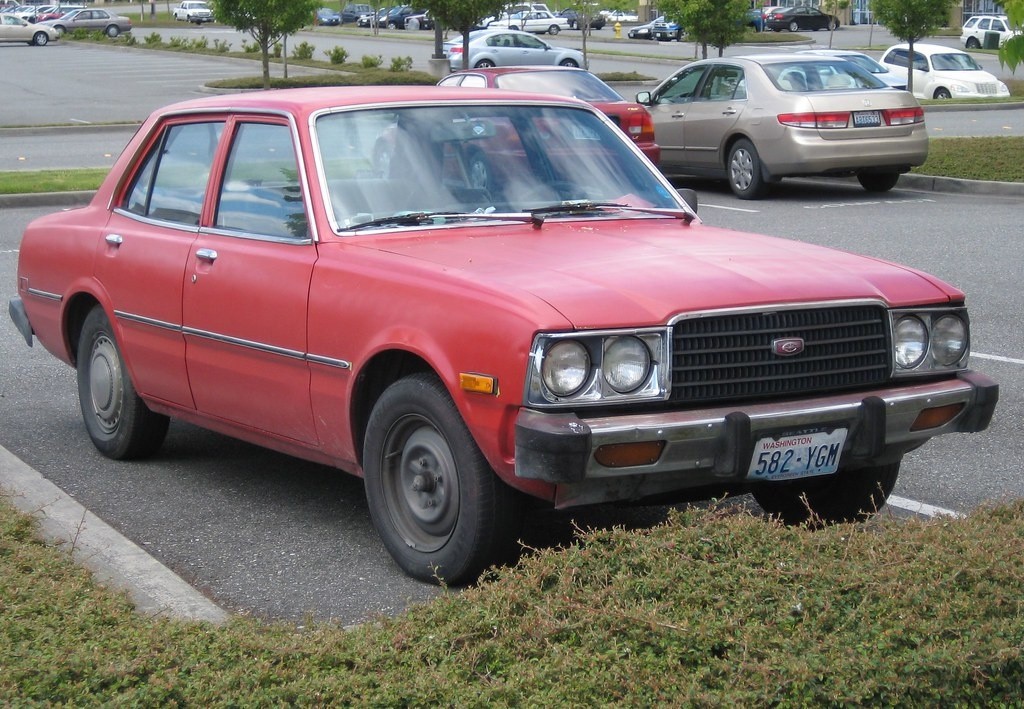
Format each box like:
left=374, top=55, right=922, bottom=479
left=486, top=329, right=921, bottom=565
left=172, top=1, right=216, bottom=25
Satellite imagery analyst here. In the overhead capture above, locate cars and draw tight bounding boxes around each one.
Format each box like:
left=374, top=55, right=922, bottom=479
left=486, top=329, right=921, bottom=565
left=8, top=87, right=998, bottom=587
left=745, top=6, right=840, bottom=32
left=878, top=41, right=1012, bottom=102
left=432, top=65, right=661, bottom=170
left=317, top=4, right=436, bottom=30
left=0, top=0, right=133, bottom=47
left=627, top=15, right=666, bottom=42
left=440, top=28, right=589, bottom=72
left=634, top=54, right=932, bottom=202
left=475, top=1, right=638, bottom=36
left=957, top=12, right=1024, bottom=51
left=796, top=48, right=908, bottom=96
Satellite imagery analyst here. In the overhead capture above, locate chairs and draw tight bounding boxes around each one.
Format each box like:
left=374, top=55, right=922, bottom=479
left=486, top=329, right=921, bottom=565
left=504, top=39, right=510, bottom=46
left=784, top=71, right=807, bottom=92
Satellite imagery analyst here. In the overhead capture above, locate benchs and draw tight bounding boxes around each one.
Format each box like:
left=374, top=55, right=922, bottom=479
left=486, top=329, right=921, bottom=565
left=185, top=176, right=427, bottom=229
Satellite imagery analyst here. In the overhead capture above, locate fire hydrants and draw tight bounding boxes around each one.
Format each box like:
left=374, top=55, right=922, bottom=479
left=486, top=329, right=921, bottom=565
left=612, top=22, right=621, bottom=41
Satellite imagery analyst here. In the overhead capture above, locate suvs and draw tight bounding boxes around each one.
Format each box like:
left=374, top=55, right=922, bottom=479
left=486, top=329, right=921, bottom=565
left=652, top=15, right=691, bottom=41
